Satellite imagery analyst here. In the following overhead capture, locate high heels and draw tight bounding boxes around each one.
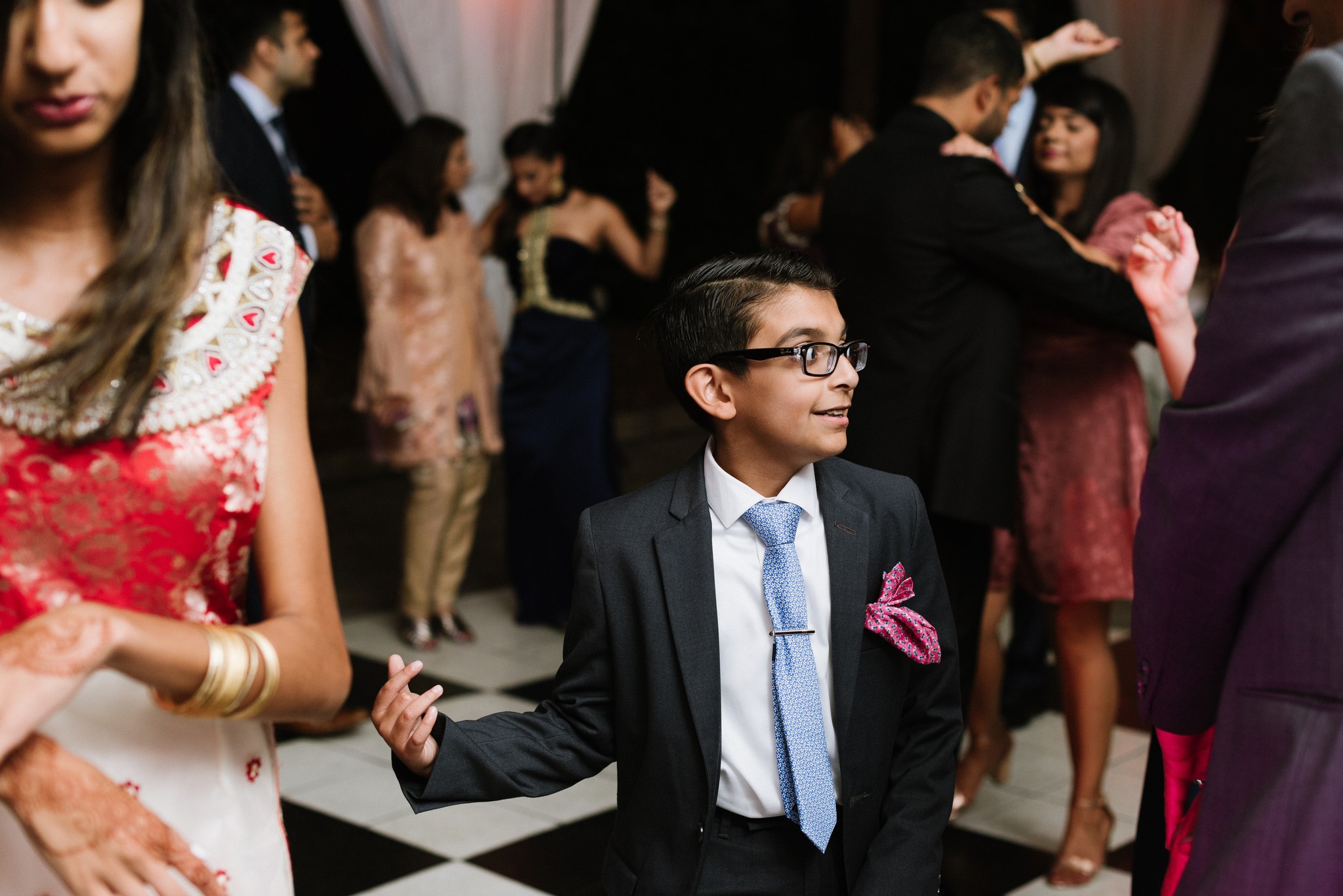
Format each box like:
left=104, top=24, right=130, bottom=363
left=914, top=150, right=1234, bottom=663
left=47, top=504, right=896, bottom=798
left=1047, top=791, right=1118, bottom=883
left=949, top=730, right=1012, bottom=819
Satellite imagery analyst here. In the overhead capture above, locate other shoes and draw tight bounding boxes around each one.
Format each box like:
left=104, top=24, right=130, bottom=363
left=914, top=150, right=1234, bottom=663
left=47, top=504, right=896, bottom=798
left=396, top=614, right=437, bottom=652
left=272, top=704, right=363, bottom=741
left=429, top=607, right=475, bottom=646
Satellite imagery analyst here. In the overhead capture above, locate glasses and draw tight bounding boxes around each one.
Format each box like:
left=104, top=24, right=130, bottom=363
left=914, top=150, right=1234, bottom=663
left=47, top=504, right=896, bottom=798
left=698, top=336, right=870, bottom=377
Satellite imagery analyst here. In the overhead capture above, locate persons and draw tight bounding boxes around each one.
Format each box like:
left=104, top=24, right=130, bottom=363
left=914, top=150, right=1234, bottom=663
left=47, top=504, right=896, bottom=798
left=1, top=0, right=355, bottom=894
left=943, top=15, right=1160, bottom=890
left=754, top=92, right=909, bottom=255
left=802, top=13, right=1167, bottom=785
left=366, top=257, right=972, bottom=896
left=1120, top=197, right=1218, bottom=872
left=463, top=116, right=681, bottom=644
left=1106, top=32, right=1343, bottom=896
left=184, top=2, right=347, bottom=637
left=351, top=109, right=508, bottom=658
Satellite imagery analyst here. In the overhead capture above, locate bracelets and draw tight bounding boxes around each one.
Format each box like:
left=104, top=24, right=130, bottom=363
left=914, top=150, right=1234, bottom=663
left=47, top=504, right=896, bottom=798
left=148, top=617, right=284, bottom=722
left=647, top=216, right=668, bottom=232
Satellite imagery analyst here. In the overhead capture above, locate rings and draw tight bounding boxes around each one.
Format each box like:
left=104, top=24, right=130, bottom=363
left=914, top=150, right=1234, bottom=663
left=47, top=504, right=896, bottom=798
left=975, top=145, right=993, bottom=159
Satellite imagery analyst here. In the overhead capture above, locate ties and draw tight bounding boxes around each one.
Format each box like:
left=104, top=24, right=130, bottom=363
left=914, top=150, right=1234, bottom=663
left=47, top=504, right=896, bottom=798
left=743, top=500, right=841, bottom=856
left=272, top=113, right=296, bottom=179
